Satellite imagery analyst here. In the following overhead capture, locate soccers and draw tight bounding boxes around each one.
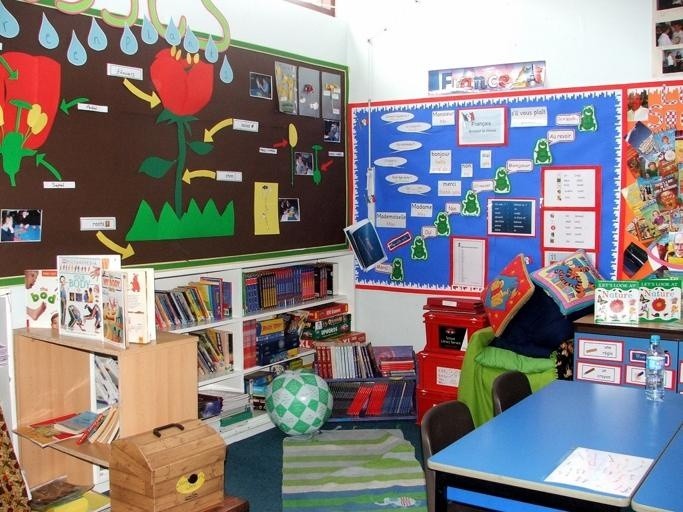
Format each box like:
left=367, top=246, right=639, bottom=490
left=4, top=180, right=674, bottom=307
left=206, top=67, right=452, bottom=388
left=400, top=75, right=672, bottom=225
left=266, top=368, right=334, bottom=438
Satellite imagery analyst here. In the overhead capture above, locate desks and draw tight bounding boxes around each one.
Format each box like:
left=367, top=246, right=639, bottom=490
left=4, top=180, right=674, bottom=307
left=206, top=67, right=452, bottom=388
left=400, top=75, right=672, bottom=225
left=427, top=378, right=682, bottom=512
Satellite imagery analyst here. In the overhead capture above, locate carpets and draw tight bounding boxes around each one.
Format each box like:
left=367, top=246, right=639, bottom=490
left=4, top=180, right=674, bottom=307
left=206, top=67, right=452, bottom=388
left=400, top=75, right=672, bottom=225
left=280, top=426, right=427, bottom=511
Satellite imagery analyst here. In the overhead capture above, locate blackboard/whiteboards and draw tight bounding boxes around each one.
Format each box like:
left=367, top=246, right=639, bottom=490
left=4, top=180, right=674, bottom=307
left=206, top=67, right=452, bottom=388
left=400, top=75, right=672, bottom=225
left=0, top=0, right=348, bottom=287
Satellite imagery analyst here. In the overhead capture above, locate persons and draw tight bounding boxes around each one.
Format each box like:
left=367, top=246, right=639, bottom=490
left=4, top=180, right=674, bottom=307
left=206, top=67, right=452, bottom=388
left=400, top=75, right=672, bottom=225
left=656, top=26, right=675, bottom=46
left=667, top=51, right=677, bottom=72
left=296, top=154, right=306, bottom=175
left=627, top=91, right=649, bottom=122
left=278, top=200, right=285, bottom=217
left=253, top=76, right=261, bottom=90
left=324, top=128, right=339, bottom=141
left=324, top=129, right=331, bottom=139
left=675, top=51, right=682, bottom=72
left=259, top=77, right=271, bottom=96
left=0, top=215, right=39, bottom=241
left=630, top=135, right=683, bottom=261
left=669, top=21, right=683, bottom=44
left=284, top=200, right=292, bottom=214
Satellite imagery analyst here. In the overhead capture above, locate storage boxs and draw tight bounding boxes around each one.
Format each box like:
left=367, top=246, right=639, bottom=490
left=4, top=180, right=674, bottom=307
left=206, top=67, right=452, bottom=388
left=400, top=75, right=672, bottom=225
left=416, top=305, right=488, bottom=428
left=108, top=418, right=226, bottom=512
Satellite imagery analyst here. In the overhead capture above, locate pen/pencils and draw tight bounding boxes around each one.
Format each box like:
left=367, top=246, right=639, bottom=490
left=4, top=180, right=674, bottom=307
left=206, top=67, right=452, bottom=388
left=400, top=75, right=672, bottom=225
left=78, top=414, right=105, bottom=444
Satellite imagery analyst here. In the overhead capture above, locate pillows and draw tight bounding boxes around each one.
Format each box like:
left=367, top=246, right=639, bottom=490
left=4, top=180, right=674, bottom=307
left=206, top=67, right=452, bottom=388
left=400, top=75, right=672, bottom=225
left=480, top=249, right=608, bottom=337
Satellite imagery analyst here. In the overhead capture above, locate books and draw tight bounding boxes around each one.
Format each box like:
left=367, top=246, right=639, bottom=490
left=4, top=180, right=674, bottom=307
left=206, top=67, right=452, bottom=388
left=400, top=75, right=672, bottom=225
left=155, top=261, right=416, bottom=429
left=26, top=480, right=95, bottom=511
left=10, top=255, right=155, bottom=446
left=46, top=488, right=110, bottom=511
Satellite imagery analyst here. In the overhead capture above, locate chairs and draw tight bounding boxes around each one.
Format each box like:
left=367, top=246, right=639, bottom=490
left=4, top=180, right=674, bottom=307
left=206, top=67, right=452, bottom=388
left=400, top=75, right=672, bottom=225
left=420, top=371, right=532, bottom=512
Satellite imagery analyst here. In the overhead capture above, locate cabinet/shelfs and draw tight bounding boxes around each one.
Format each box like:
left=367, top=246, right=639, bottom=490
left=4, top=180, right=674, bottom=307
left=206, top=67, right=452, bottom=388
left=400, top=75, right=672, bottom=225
left=151, top=248, right=353, bottom=444
left=12, top=319, right=198, bottom=512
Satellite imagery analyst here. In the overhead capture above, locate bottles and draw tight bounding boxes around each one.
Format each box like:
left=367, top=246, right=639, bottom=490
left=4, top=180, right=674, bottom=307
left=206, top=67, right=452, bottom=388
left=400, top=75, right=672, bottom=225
left=644, top=335, right=667, bottom=402
